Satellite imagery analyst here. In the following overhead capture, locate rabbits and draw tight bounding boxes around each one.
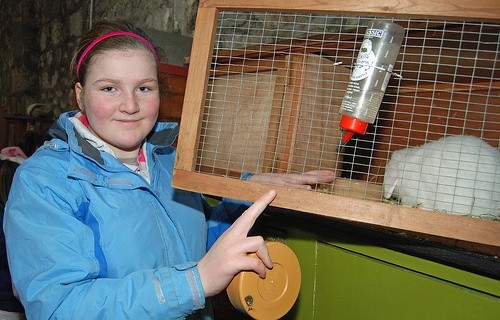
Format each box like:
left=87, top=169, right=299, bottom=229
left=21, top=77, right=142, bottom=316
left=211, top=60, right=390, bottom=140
left=382, top=134, right=500, bottom=221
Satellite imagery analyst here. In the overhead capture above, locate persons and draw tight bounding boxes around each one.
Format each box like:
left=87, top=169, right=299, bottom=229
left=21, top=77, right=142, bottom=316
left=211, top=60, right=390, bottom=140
left=2, top=21, right=338, bottom=320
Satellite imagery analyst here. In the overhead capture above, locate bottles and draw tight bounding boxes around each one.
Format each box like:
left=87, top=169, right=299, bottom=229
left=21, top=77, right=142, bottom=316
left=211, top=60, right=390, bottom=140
left=339, top=18, right=405, bottom=143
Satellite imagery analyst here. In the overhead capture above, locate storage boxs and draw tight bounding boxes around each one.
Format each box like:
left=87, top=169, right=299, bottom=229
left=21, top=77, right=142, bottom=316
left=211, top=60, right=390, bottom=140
left=364, top=79, right=500, bottom=185
left=196, top=50, right=353, bottom=187
